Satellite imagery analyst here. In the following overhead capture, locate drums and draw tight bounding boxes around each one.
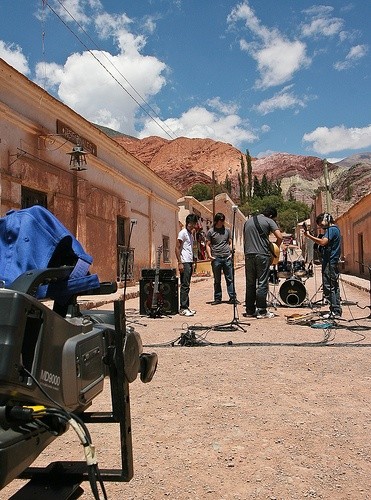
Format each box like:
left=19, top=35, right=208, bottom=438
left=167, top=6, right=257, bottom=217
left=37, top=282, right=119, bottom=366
left=293, top=260, right=307, bottom=276
left=292, top=268, right=313, bottom=278
left=268, top=269, right=280, bottom=286
left=277, top=260, right=293, bottom=279
left=287, top=275, right=303, bottom=282
left=279, top=279, right=307, bottom=307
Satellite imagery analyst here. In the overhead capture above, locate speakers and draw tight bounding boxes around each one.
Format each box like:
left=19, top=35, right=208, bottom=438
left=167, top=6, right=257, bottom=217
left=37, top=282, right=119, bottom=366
left=140, top=276, right=179, bottom=315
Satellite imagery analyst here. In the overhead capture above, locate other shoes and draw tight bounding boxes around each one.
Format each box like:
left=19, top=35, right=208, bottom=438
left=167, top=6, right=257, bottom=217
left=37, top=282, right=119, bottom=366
left=229, top=298, right=239, bottom=303
left=323, top=312, right=340, bottom=318
left=211, top=301, right=221, bottom=305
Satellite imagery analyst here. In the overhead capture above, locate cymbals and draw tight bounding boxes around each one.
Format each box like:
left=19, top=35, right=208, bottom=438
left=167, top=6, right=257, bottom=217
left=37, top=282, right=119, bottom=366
left=281, top=244, right=300, bottom=249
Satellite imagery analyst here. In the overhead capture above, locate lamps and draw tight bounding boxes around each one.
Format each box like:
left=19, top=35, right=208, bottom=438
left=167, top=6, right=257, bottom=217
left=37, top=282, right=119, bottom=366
left=34, top=133, right=88, bottom=172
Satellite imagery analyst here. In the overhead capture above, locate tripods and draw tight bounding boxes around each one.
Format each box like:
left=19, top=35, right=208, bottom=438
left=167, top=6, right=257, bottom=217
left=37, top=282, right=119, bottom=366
left=309, top=218, right=347, bottom=326
left=213, top=212, right=250, bottom=333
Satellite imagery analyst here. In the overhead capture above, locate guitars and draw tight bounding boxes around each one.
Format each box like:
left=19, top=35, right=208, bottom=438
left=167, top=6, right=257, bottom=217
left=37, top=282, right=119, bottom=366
left=269, top=242, right=280, bottom=266
left=147, top=244, right=165, bottom=313
left=302, top=221, right=325, bottom=265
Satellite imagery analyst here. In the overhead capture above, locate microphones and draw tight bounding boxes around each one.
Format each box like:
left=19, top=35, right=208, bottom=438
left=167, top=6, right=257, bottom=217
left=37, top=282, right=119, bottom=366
left=324, top=214, right=331, bottom=221
left=232, top=205, right=238, bottom=212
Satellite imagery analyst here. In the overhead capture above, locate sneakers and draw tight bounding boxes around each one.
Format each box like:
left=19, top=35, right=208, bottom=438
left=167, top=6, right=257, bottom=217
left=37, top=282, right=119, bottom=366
left=257, top=312, right=274, bottom=318
left=190, top=311, right=196, bottom=314
left=246, top=309, right=257, bottom=316
left=180, top=308, right=194, bottom=316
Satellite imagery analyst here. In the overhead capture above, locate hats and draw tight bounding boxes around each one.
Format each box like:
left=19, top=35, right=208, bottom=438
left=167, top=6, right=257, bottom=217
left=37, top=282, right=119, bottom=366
left=0, top=205, right=100, bottom=300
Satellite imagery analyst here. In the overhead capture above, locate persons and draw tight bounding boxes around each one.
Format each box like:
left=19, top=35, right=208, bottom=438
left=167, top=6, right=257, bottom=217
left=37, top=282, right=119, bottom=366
left=206, top=213, right=240, bottom=305
left=305, top=213, right=342, bottom=318
left=243, top=207, right=282, bottom=319
left=175, top=214, right=198, bottom=317
